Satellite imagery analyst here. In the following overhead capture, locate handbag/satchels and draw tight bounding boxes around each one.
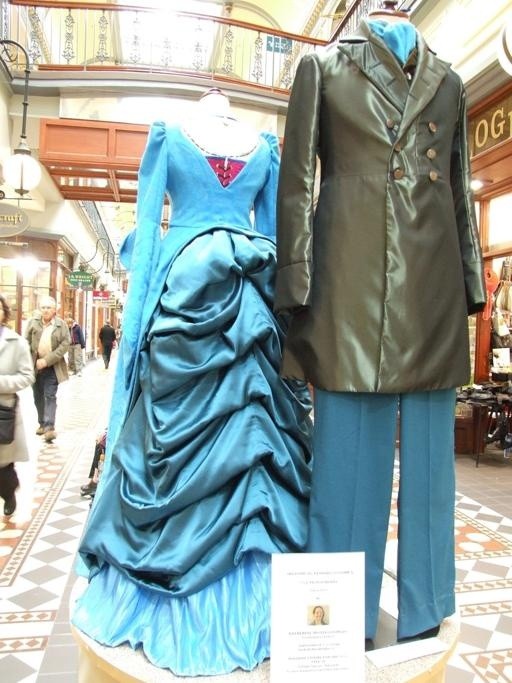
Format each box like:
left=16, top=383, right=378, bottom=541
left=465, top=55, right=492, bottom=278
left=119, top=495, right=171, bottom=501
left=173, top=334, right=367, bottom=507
left=0, top=405, right=16, bottom=444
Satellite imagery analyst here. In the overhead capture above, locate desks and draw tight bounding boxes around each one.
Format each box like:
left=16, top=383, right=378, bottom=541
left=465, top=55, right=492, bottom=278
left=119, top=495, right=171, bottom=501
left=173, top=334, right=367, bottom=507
left=455, top=391, right=511, bottom=467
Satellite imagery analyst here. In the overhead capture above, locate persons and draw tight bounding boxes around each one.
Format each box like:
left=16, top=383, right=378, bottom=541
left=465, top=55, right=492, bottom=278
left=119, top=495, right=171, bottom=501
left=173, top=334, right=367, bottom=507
left=84, top=85, right=316, bottom=671
left=289, top=3, right=488, bottom=651
left=310, top=606, right=327, bottom=625
left=81, top=433, right=108, bottom=492
left=99, top=319, right=117, bottom=369
left=1, top=294, right=36, bottom=513
left=66, top=316, right=86, bottom=376
left=26, top=294, right=73, bottom=442
left=115, top=325, right=121, bottom=345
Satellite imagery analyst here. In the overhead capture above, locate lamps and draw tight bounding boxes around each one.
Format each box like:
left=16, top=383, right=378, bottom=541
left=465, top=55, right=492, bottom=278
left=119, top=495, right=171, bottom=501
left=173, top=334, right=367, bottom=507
left=1, top=37, right=42, bottom=196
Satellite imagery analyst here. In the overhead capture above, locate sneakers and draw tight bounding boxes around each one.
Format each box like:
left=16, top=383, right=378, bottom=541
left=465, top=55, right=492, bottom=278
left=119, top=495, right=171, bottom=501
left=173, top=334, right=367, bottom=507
left=3, top=494, right=17, bottom=515
left=35, top=426, right=58, bottom=443
